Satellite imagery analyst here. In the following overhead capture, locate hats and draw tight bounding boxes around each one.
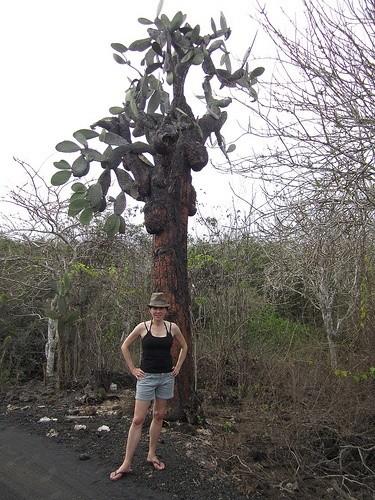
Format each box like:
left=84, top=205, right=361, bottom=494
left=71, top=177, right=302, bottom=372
left=147, top=292, right=170, bottom=307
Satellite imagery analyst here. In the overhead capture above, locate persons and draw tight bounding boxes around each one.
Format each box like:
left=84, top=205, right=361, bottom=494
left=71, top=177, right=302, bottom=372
left=109, top=293, right=187, bottom=481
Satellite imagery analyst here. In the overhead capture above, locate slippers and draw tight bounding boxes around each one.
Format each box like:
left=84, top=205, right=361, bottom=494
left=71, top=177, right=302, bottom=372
left=109, top=469, right=125, bottom=481
left=148, top=461, right=166, bottom=471
